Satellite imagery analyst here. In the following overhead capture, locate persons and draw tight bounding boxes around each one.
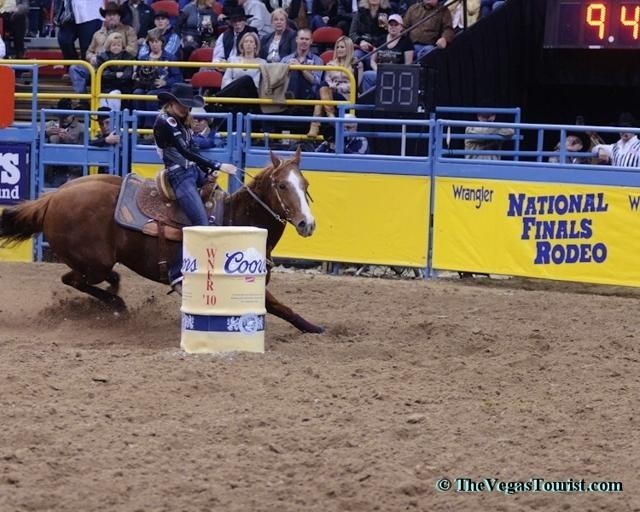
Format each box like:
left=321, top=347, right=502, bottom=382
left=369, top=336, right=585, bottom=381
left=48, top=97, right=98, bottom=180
left=0, top=0, right=639, bottom=294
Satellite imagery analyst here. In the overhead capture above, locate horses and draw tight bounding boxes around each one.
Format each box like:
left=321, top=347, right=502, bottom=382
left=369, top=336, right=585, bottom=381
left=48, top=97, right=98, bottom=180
left=0, top=146, right=328, bottom=335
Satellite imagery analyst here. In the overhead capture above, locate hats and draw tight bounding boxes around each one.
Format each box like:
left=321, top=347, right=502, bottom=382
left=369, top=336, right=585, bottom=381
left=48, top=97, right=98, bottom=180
left=188, top=106, right=209, bottom=121
left=221, top=5, right=255, bottom=21
left=50, top=96, right=81, bottom=119
left=609, top=109, right=639, bottom=129
left=387, top=13, right=403, bottom=25
left=158, top=83, right=205, bottom=110
left=564, top=129, right=591, bottom=154
left=93, top=104, right=115, bottom=122
left=152, top=10, right=170, bottom=19
left=99, top=1, right=128, bottom=20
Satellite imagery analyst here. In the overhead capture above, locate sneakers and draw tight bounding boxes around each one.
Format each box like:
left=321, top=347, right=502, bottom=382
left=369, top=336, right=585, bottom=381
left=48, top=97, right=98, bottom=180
left=168, top=269, right=183, bottom=299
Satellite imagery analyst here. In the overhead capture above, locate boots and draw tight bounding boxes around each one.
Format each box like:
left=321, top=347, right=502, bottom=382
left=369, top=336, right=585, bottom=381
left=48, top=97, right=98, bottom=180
left=307, top=86, right=336, bottom=137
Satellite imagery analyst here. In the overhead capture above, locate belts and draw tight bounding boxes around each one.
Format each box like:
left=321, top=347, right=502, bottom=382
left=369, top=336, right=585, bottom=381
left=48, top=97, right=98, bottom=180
left=163, top=158, right=195, bottom=175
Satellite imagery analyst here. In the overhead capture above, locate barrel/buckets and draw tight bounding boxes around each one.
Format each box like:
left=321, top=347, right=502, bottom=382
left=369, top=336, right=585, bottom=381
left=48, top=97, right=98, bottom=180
left=179, top=225, right=269, bottom=354
